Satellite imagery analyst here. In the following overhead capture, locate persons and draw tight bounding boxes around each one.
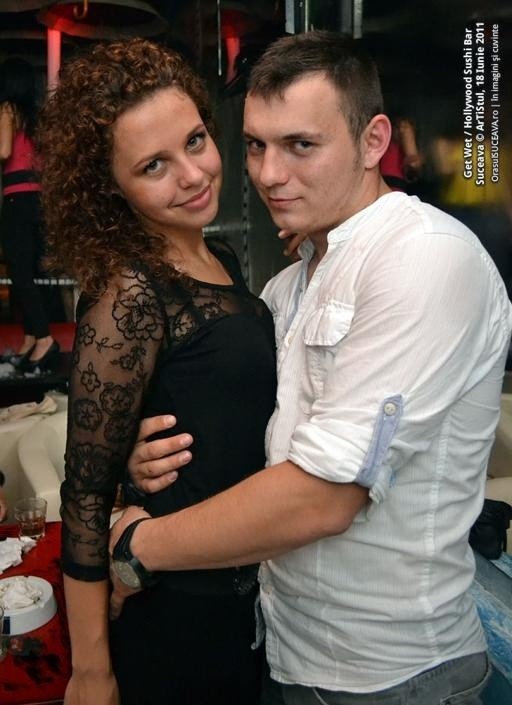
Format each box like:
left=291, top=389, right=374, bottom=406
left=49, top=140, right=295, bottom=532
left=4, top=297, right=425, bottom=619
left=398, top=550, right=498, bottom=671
left=370, top=66, right=425, bottom=198
left=34, top=33, right=312, bottom=704
left=0, top=42, right=62, bottom=372
left=102, top=29, right=512, bottom=703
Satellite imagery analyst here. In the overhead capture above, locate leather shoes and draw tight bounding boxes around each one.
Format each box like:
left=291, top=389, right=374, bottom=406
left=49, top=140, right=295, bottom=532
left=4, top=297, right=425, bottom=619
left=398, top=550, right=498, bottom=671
left=11, top=341, right=63, bottom=373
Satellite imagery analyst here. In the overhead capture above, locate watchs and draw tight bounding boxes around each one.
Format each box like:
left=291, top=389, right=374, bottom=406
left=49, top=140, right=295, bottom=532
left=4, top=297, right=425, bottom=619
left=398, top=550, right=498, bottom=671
left=110, top=516, right=166, bottom=593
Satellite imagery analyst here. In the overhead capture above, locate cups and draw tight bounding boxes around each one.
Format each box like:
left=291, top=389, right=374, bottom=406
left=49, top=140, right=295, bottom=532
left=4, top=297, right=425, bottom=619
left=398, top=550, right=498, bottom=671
left=0, top=605, right=8, bottom=661
left=13, top=498, right=48, bottom=542
